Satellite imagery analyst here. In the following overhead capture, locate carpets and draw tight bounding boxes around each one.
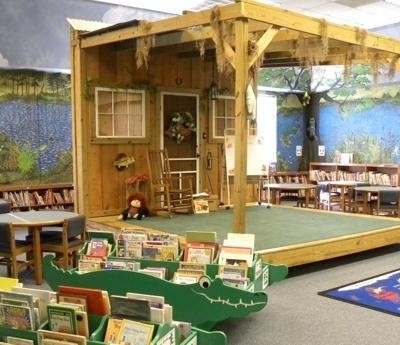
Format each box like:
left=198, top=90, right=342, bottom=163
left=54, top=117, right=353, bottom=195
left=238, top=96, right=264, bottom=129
left=119, top=205, right=400, bottom=251
left=318, top=267, right=400, bottom=318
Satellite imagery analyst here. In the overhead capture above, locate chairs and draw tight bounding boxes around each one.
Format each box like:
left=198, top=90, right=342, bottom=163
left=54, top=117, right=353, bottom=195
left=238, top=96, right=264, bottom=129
left=146, top=147, right=195, bottom=218
left=0, top=201, right=86, bottom=286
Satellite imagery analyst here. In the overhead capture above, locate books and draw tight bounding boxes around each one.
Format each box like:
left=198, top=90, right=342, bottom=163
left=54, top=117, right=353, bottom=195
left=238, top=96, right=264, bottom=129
left=3, top=188, right=72, bottom=210
left=0, top=228, right=253, bottom=345
left=277, top=169, right=398, bottom=198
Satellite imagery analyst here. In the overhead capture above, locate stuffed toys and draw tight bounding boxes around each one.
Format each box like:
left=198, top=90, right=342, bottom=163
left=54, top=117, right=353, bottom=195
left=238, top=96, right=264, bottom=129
left=118, top=192, right=149, bottom=220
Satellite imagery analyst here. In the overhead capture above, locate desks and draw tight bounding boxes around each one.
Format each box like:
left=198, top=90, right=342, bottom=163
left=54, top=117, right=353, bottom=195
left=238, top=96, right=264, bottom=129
left=262, top=181, right=400, bottom=216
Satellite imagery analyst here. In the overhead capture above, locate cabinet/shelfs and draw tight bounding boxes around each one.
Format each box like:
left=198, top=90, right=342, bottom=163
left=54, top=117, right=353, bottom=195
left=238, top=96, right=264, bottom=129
left=268, top=162, right=399, bottom=203
left=0, top=183, right=75, bottom=212
